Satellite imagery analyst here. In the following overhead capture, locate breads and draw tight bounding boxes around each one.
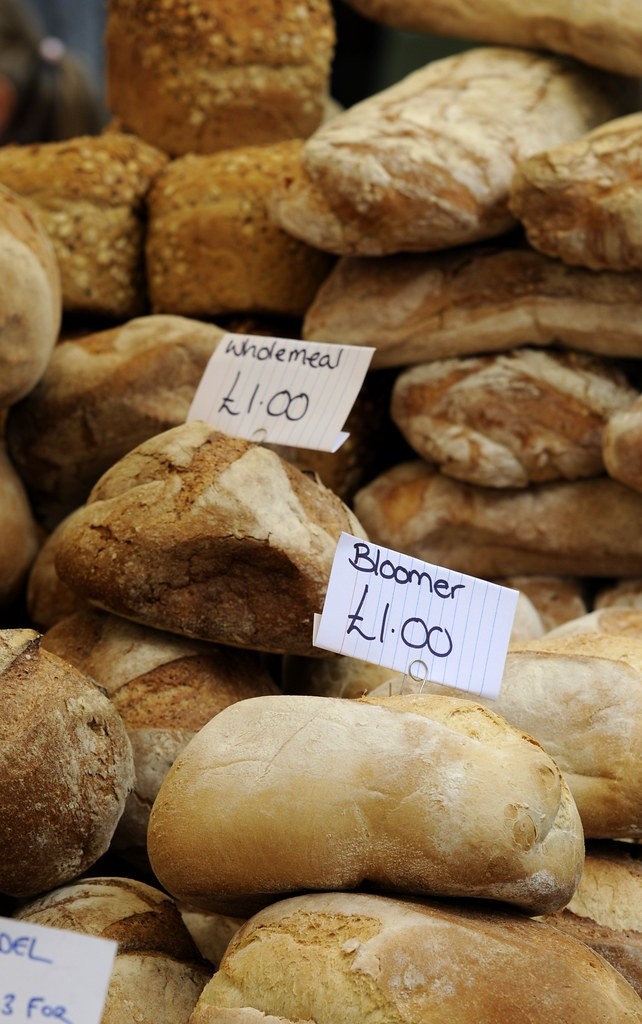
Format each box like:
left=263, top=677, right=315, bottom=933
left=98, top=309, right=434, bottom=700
left=0, top=0, right=642, bottom=1024
